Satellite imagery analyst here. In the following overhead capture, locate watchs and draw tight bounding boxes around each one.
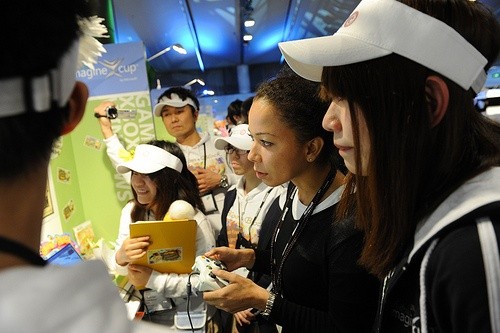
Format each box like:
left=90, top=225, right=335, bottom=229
left=218, top=176, right=228, bottom=187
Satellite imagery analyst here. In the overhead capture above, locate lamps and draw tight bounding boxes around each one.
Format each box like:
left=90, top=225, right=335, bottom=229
left=184, top=78, right=205, bottom=87
left=243, top=13, right=255, bottom=27
left=147, top=43, right=188, bottom=62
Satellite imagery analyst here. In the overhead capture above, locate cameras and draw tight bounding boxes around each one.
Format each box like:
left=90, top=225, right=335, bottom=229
left=104, top=106, right=137, bottom=120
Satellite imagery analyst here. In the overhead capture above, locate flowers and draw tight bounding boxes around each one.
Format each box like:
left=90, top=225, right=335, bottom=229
left=77, top=14, right=110, bottom=72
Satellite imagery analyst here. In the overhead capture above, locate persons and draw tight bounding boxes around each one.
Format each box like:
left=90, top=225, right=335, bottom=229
left=0, top=0, right=137, bottom=333
left=94, top=0, right=500, bottom=333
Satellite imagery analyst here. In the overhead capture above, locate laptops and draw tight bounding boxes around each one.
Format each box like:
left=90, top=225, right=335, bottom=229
left=42, top=243, right=86, bottom=267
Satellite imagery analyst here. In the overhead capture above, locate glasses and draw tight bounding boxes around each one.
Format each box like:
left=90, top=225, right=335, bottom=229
left=223, top=146, right=252, bottom=155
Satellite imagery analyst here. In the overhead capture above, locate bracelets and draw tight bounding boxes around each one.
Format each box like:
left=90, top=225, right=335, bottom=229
left=261, top=289, right=276, bottom=317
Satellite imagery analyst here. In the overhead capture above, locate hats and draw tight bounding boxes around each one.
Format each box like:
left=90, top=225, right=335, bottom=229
left=214, top=124, right=254, bottom=150
left=154, top=93, right=198, bottom=117
left=116, top=144, right=184, bottom=175
left=277, top=0, right=490, bottom=96
